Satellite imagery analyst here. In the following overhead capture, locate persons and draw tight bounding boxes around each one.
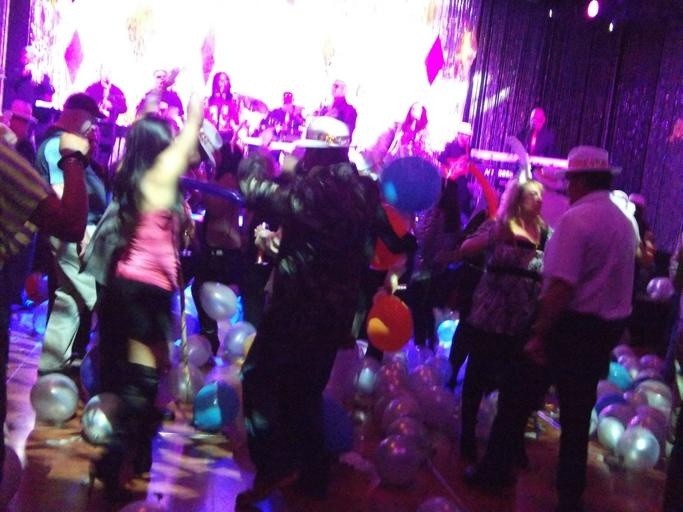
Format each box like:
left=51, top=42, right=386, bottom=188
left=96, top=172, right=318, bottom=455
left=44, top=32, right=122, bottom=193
left=1, top=55, right=683, bottom=512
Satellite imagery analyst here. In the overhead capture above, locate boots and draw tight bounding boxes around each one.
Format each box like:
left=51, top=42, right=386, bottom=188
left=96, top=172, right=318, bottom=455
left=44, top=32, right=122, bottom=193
left=85, top=362, right=157, bottom=502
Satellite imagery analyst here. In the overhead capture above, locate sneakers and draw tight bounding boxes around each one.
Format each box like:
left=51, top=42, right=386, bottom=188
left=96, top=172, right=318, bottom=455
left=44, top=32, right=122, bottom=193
left=446, top=375, right=458, bottom=388
left=458, top=433, right=477, bottom=459
left=518, top=444, right=528, bottom=465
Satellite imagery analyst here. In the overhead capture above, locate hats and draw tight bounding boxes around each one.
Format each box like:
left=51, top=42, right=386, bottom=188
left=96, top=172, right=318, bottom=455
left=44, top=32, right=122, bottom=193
left=198, top=119, right=224, bottom=165
left=65, top=92, right=107, bottom=118
left=11, top=101, right=37, bottom=123
left=458, top=122, right=472, bottom=135
left=555, top=146, right=624, bottom=175
left=298, top=117, right=350, bottom=149
